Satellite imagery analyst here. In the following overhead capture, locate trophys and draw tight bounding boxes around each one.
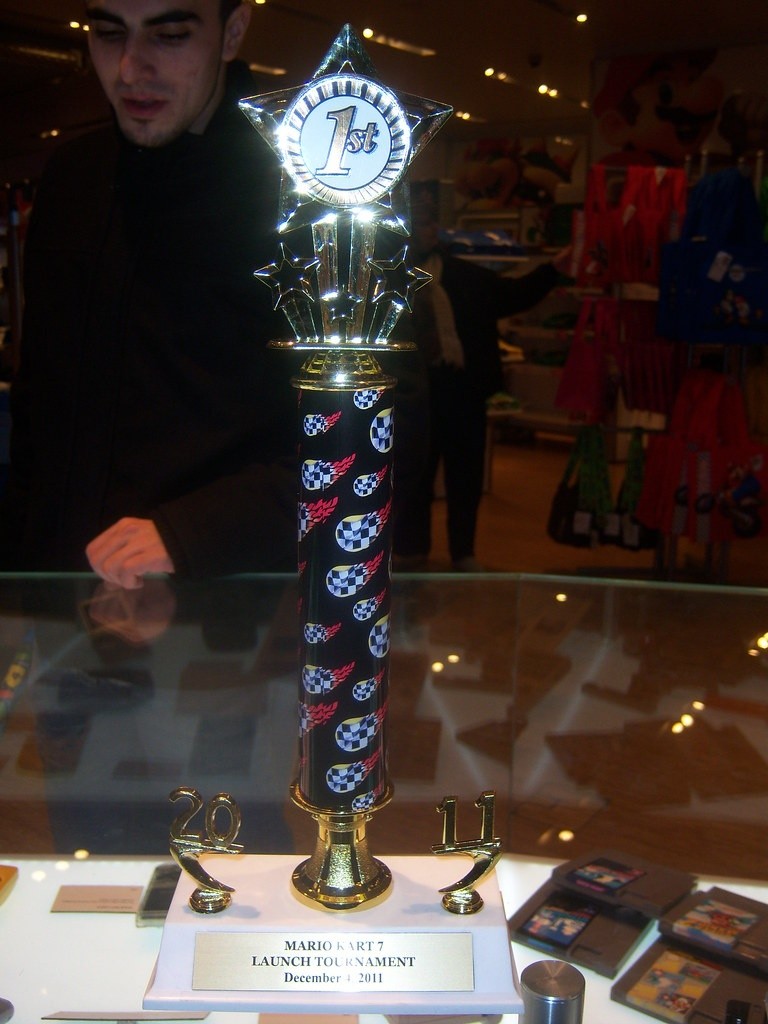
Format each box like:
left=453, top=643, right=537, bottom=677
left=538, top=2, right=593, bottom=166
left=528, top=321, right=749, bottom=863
left=167, top=22, right=528, bottom=1016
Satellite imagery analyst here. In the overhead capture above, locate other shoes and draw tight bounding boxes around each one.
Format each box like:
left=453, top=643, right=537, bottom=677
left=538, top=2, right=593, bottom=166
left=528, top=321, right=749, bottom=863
left=450, top=553, right=476, bottom=573
left=396, top=551, right=429, bottom=569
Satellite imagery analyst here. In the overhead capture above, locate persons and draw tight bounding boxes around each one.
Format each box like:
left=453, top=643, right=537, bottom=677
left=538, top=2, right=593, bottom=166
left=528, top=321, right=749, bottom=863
left=0, top=0, right=305, bottom=774
left=394, top=191, right=572, bottom=573
